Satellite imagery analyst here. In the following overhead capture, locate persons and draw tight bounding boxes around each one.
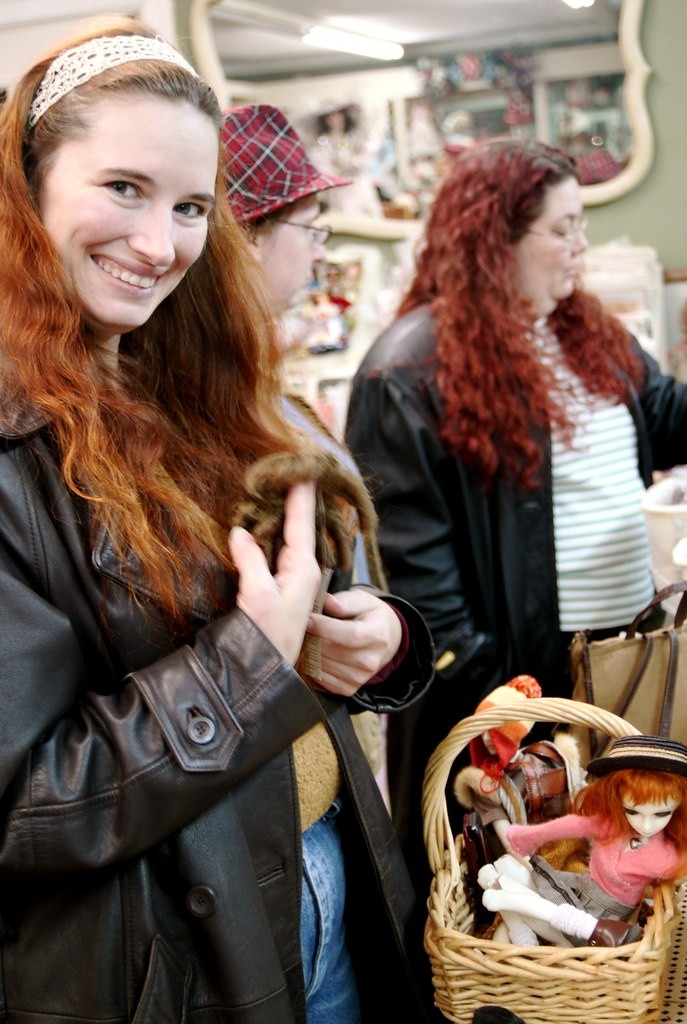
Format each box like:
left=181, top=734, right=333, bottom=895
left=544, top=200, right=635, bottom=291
left=308, top=101, right=385, bottom=223
left=347, top=135, right=687, bottom=919
left=0, top=16, right=445, bottom=1024
left=217, top=103, right=355, bottom=315
left=478, top=735, right=687, bottom=952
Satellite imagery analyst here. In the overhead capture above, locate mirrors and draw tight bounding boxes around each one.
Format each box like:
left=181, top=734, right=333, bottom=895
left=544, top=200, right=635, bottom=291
left=210, top=2, right=631, bottom=220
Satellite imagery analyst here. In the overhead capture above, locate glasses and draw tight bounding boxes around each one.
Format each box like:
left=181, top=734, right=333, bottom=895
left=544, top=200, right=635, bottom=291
left=263, top=218, right=332, bottom=244
left=514, top=220, right=589, bottom=243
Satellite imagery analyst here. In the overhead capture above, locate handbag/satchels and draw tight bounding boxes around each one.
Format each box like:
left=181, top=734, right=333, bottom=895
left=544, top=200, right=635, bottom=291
left=566, top=576, right=687, bottom=769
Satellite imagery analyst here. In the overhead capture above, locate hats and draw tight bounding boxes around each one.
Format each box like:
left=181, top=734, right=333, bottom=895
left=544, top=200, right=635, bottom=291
left=214, top=104, right=355, bottom=226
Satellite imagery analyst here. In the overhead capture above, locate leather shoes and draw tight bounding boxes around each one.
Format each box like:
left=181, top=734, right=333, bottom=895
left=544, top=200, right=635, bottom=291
left=588, top=917, right=643, bottom=963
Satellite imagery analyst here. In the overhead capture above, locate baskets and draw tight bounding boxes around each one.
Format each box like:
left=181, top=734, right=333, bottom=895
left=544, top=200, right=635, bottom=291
left=419, top=697, right=685, bottom=1024
